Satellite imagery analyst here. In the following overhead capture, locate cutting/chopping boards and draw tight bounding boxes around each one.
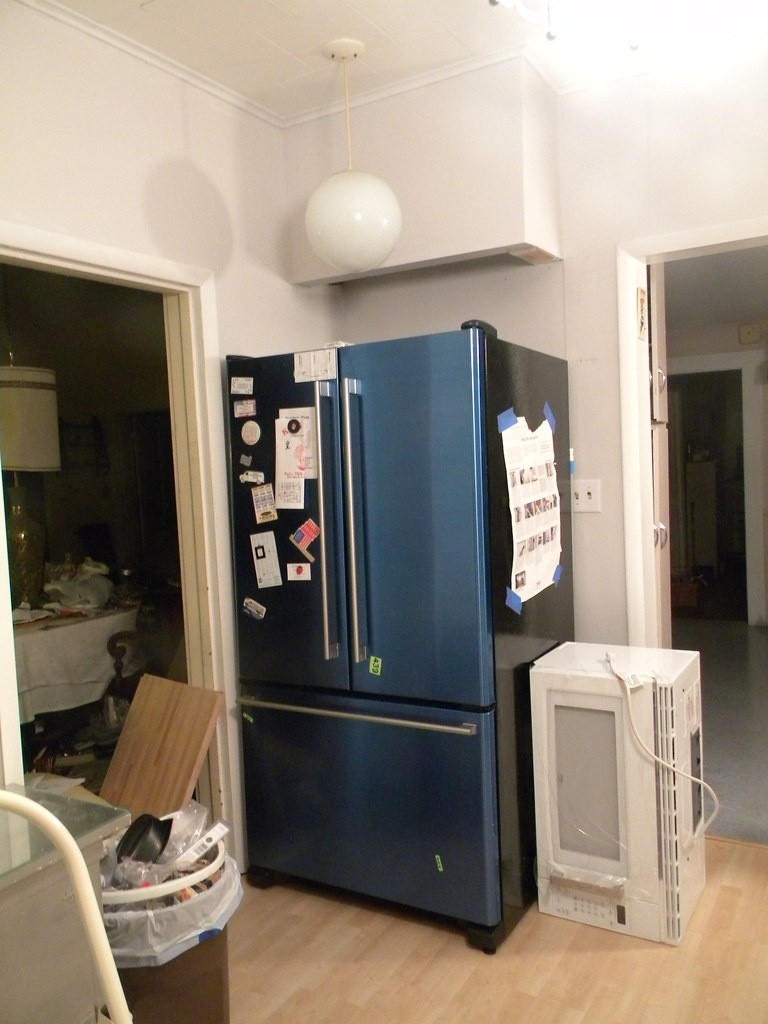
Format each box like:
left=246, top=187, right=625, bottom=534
left=98, top=673, right=227, bottom=825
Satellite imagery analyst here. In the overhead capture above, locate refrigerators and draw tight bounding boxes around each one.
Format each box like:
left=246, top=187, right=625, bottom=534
left=222, top=319, right=574, bottom=956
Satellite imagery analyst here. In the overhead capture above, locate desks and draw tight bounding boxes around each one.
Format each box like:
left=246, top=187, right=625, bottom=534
left=16, top=602, right=137, bottom=726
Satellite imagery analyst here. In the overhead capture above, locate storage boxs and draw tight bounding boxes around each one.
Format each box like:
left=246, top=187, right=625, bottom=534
left=101, top=817, right=240, bottom=1024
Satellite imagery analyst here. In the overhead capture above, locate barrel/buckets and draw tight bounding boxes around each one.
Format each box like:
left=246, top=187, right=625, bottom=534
left=100, top=831, right=229, bottom=1024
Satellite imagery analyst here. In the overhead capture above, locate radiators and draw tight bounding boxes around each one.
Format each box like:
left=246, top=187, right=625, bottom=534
left=530, top=640, right=719, bottom=947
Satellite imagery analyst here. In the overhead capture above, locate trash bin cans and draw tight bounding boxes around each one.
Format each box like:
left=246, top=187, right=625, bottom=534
left=88, top=828, right=244, bottom=1024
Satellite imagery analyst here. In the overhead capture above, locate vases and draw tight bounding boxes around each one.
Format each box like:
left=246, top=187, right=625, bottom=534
left=5, top=485, right=47, bottom=610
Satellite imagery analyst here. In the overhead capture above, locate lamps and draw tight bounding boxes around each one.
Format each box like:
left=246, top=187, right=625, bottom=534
left=303, top=36, right=401, bottom=274
left=0, top=267, right=61, bottom=474
